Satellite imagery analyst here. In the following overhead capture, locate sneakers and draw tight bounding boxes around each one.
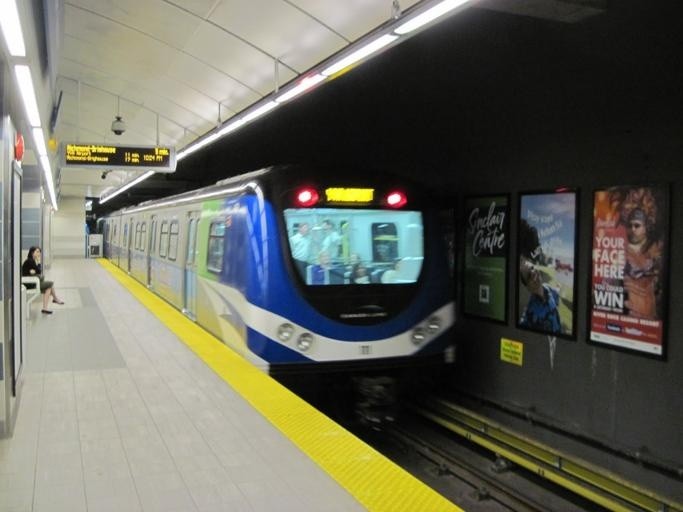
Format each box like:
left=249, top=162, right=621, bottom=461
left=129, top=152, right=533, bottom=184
left=42, top=310, right=52, bottom=314
left=53, top=300, right=64, bottom=304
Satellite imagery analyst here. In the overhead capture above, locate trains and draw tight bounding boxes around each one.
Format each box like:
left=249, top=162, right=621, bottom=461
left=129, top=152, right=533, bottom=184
left=100, top=150, right=478, bottom=426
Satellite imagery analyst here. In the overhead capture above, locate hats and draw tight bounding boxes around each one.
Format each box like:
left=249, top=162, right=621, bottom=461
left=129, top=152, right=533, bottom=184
left=629, top=209, right=646, bottom=224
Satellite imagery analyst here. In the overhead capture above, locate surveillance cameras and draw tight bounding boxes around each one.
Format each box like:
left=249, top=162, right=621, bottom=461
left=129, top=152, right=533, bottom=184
left=111, top=115, right=126, bottom=135
left=102, top=171, right=106, bottom=179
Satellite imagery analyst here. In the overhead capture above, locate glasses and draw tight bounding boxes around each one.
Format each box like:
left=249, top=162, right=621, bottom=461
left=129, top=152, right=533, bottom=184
left=628, top=223, right=640, bottom=227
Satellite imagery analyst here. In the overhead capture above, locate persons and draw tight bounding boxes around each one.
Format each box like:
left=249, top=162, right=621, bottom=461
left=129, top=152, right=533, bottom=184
left=392, top=258, right=401, bottom=270
left=529, top=247, right=546, bottom=267
left=19, top=246, right=65, bottom=315
left=517, top=259, right=565, bottom=333
left=311, top=250, right=332, bottom=285
left=287, top=222, right=318, bottom=285
left=348, top=263, right=372, bottom=285
left=605, top=185, right=663, bottom=319
left=311, top=219, right=345, bottom=261
left=348, top=252, right=360, bottom=265
